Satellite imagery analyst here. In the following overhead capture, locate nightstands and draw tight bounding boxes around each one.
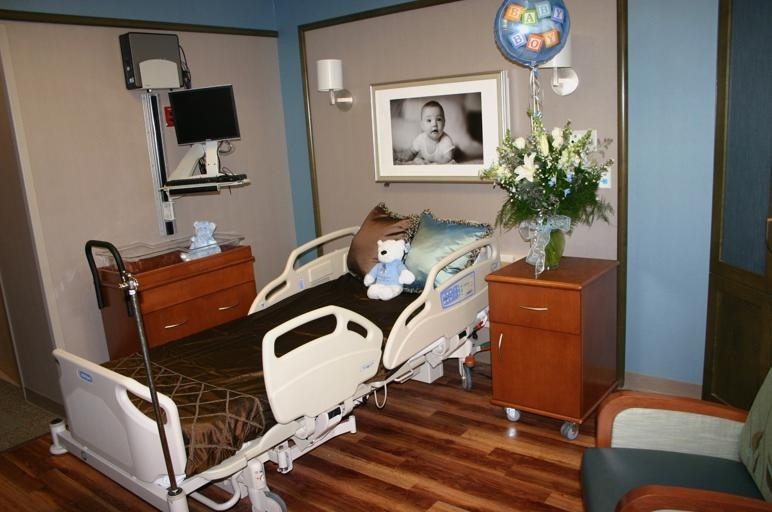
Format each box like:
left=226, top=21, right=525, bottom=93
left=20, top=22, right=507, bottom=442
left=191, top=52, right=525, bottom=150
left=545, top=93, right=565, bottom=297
left=486, top=254, right=620, bottom=424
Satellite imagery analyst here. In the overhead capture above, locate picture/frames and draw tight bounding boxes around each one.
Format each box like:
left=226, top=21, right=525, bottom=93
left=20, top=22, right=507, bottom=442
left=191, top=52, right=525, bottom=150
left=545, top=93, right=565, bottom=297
left=368, top=70, right=509, bottom=187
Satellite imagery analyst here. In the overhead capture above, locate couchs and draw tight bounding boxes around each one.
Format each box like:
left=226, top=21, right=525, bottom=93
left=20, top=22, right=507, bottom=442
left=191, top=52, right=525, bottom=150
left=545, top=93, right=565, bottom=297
left=579, top=363, right=772, bottom=512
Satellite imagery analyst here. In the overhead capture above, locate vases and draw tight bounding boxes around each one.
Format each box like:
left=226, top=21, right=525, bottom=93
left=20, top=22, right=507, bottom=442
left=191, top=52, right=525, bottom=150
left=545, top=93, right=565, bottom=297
left=540, top=221, right=566, bottom=273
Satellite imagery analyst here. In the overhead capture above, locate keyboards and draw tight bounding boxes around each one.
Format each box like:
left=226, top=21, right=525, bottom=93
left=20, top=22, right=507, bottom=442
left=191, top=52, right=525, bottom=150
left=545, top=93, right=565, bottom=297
left=163, top=174, right=247, bottom=187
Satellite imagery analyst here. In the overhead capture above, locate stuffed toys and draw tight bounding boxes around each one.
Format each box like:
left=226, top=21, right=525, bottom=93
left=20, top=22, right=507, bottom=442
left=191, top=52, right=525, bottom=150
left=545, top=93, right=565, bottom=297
left=363, top=238, right=416, bottom=300
left=180, top=220, right=221, bottom=262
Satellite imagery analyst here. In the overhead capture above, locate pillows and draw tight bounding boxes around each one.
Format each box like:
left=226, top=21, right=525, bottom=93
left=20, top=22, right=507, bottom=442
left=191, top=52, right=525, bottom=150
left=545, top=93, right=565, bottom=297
left=409, top=208, right=493, bottom=296
left=347, top=205, right=417, bottom=284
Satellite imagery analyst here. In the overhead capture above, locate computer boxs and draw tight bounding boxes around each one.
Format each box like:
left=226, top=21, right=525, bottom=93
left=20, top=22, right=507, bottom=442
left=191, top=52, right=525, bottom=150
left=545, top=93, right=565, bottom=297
left=118, top=31, right=184, bottom=90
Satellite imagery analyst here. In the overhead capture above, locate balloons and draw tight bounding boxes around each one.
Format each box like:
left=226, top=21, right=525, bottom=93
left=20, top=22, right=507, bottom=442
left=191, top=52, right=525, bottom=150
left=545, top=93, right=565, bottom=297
left=493, top=0, right=571, bottom=119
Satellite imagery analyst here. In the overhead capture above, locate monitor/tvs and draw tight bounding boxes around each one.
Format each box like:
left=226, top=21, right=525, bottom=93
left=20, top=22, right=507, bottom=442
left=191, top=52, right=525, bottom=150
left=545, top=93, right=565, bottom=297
left=167, top=83, right=243, bottom=176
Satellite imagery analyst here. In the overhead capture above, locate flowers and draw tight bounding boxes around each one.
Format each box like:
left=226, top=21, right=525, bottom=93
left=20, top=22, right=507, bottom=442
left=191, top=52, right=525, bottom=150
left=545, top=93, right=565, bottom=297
left=478, top=108, right=616, bottom=235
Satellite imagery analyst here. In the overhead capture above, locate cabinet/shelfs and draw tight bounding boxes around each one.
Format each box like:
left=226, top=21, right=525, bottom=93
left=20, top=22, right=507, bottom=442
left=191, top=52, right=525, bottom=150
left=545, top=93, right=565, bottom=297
left=98, top=244, right=257, bottom=359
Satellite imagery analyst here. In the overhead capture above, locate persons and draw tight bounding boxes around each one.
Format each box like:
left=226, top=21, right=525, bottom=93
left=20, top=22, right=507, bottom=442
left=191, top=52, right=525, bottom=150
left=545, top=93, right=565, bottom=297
left=398, top=100, right=455, bottom=165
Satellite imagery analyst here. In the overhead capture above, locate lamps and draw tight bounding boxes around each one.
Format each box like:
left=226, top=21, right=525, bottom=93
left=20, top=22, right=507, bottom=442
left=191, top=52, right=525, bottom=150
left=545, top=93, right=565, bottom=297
left=313, top=58, right=354, bottom=111
left=532, top=29, right=577, bottom=96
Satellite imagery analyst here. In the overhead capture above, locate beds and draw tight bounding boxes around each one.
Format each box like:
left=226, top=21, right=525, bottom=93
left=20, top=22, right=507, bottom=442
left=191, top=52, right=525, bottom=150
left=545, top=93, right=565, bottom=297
left=49, top=217, right=499, bottom=512
